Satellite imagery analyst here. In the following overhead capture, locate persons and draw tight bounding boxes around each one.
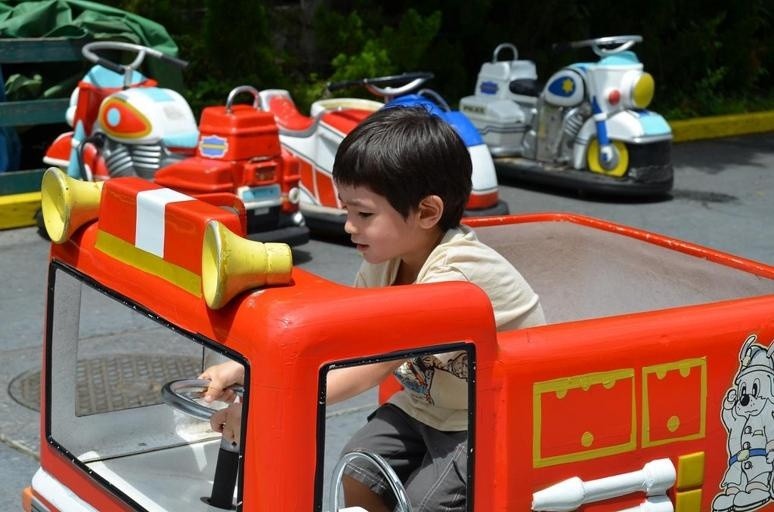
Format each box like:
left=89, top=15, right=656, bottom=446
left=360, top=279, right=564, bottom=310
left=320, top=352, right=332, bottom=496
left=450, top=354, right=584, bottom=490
left=189, top=103, right=544, bottom=512
